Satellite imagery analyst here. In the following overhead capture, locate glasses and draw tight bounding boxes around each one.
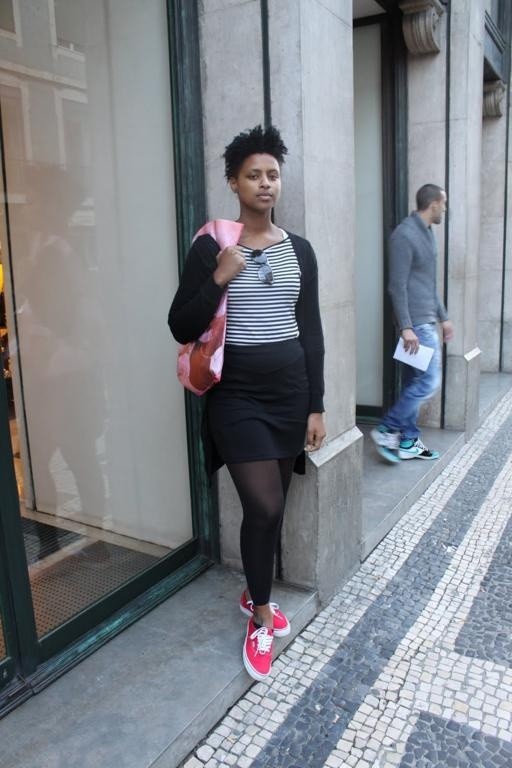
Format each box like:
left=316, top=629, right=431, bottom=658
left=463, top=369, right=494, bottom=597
left=251, top=248, right=275, bottom=285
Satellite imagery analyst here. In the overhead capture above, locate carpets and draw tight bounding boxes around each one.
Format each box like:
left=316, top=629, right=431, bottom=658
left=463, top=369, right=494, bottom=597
left=0, top=515, right=160, bottom=661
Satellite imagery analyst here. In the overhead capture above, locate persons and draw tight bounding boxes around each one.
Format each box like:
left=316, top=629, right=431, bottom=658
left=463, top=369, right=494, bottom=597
left=369, top=182, right=455, bottom=463
left=165, top=123, right=329, bottom=681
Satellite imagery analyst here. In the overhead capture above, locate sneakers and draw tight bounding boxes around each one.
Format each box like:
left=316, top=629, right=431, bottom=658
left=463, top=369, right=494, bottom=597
left=239, top=588, right=291, bottom=639
left=397, top=439, right=441, bottom=461
left=242, top=614, right=273, bottom=683
left=369, top=424, right=402, bottom=464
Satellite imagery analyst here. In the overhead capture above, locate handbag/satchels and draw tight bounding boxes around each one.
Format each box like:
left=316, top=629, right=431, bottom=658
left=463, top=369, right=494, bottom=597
left=175, top=217, right=245, bottom=398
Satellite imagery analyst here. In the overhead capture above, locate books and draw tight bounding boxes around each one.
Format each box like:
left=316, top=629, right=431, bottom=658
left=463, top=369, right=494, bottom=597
left=393, top=337, right=435, bottom=373
